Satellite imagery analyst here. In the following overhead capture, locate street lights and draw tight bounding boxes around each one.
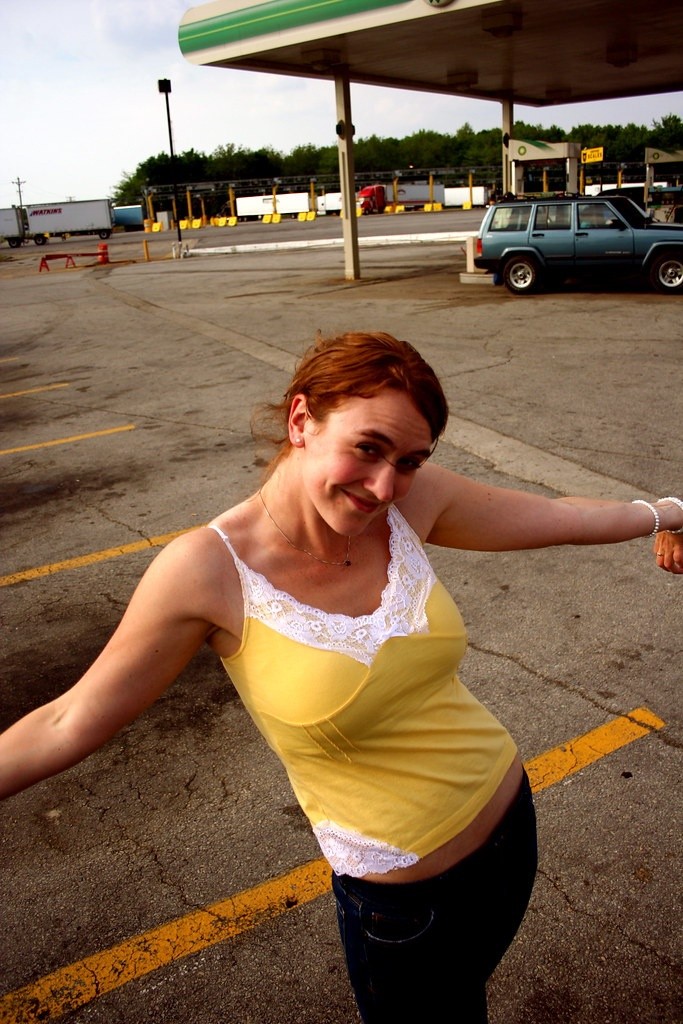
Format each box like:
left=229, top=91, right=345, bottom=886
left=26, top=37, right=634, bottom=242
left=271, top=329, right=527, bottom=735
left=158, top=78, right=183, bottom=257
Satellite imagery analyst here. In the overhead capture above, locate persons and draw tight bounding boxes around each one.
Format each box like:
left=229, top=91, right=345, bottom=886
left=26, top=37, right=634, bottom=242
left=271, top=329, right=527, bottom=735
left=0, top=333, right=683, bottom=1024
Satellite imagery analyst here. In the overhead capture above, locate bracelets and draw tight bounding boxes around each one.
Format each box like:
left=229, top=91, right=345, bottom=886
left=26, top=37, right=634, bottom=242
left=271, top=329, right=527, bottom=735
left=633, top=500, right=659, bottom=538
left=661, top=497, right=683, bottom=533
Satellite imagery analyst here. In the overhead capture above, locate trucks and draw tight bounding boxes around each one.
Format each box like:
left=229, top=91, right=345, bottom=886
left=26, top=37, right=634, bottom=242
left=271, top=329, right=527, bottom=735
left=0, top=198, right=145, bottom=247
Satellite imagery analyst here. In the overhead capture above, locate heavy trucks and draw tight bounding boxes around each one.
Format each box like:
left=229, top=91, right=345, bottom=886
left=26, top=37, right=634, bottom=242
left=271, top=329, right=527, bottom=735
left=443, top=187, right=489, bottom=208
left=357, top=185, right=445, bottom=215
left=216, top=191, right=310, bottom=222
left=316, top=192, right=342, bottom=216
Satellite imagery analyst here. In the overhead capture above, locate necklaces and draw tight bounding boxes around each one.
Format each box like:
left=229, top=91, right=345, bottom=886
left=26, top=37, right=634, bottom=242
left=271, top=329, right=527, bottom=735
left=253, top=487, right=352, bottom=571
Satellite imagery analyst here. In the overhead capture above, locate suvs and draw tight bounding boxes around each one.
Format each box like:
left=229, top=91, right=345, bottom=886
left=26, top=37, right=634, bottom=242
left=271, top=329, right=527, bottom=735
left=472, top=195, right=683, bottom=301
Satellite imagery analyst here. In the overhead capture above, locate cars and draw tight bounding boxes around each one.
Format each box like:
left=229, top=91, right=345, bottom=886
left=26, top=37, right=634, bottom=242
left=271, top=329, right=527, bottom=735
left=597, top=186, right=683, bottom=224
